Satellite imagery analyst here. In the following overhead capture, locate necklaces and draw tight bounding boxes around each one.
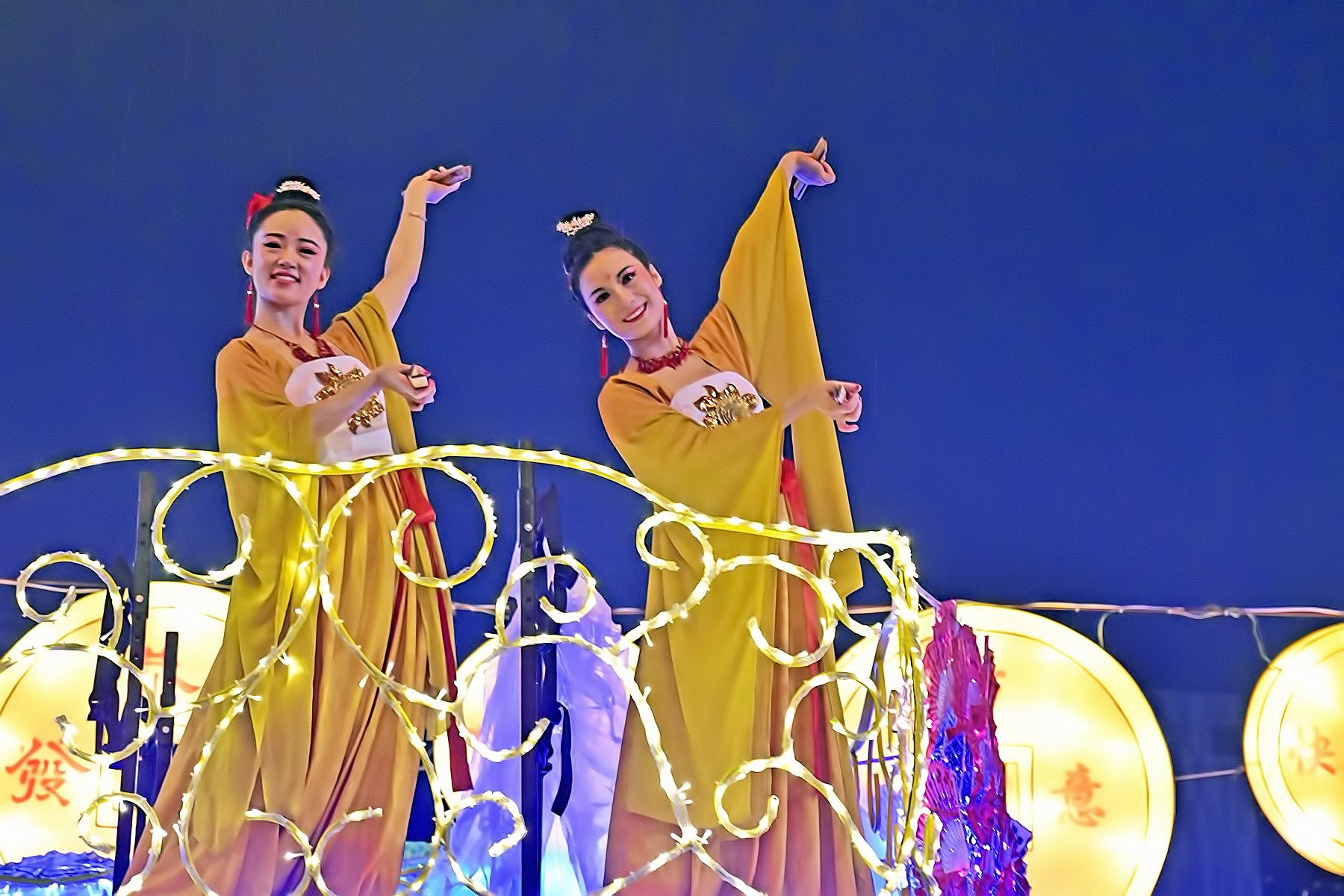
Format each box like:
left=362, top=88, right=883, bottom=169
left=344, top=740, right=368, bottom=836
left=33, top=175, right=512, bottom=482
left=630, top=337, right=694, bottom=374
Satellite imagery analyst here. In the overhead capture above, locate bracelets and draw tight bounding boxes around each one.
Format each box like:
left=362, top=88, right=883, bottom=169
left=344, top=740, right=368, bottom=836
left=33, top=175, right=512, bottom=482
left=410, top=213, right=427, bottom=222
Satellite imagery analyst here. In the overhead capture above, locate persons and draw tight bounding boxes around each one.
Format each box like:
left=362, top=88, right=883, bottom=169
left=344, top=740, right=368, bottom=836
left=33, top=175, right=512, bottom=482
left=121, top=166, right=460, bottom=896
left=556, top=152, right=863, bottom=896
